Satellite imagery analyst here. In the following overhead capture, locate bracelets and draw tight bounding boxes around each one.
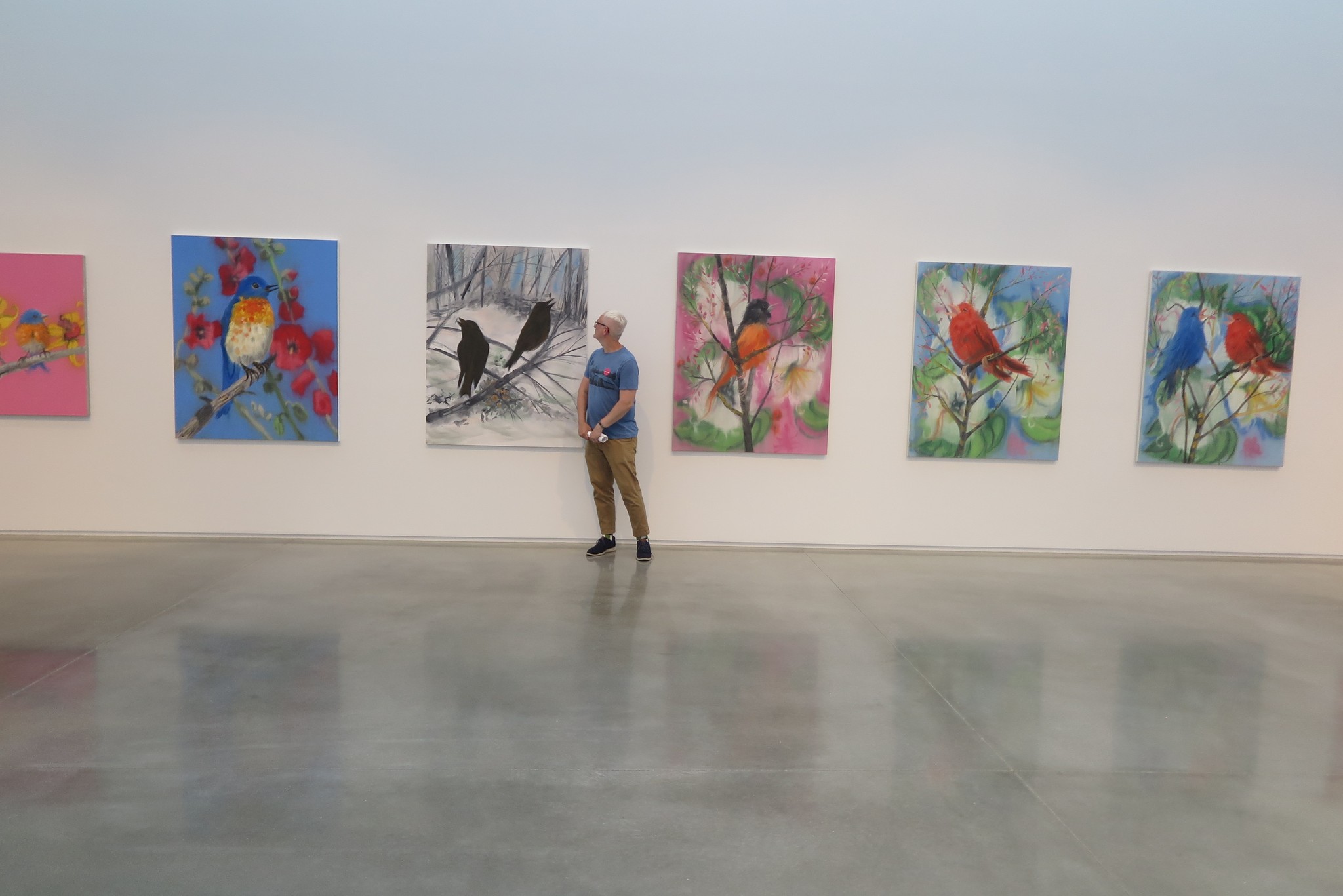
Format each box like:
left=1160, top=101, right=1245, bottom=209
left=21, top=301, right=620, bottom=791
left=598, top=421, right=606, bottom=429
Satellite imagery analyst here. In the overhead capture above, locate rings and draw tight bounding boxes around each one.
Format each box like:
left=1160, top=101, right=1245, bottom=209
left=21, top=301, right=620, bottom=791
left=593, top=441, right=595, bottom=442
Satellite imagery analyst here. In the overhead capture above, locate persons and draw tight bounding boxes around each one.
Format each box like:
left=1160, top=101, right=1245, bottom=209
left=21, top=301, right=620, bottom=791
left=577, top=310, right=652, bottom=562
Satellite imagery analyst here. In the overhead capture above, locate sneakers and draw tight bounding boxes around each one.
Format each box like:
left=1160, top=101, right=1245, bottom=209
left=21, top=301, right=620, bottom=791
left=586, top=535, right=616, bottom=556
left=637, top=537, right=652, bottom=561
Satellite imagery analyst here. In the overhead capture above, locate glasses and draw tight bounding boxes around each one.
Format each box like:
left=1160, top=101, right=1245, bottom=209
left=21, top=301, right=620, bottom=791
left=594, top=320, right=610, bottom=334
